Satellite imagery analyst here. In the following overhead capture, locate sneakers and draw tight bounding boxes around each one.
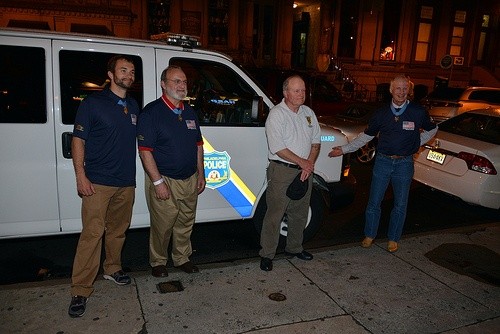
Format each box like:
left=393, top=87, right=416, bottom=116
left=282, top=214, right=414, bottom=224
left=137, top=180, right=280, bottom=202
left=68, top=295, right=89, bottom=318
left=102, top=270, right=131, bottom=284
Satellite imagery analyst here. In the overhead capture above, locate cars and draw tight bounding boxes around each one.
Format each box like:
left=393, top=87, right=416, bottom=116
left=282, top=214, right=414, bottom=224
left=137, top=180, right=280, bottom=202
left=413, top=106, right=500, bottom=211
left=317, top=104, right=379, bottom=163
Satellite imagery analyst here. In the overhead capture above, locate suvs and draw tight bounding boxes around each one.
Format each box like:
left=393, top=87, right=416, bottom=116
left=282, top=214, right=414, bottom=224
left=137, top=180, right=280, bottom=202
left=419, top=85, right=499, bottom=122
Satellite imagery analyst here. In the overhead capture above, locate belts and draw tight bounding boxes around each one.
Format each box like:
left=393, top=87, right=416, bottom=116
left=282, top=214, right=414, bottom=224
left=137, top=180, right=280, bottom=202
left=271, top=159, right=303, bottom=171
left=384, top=155, right=406, bottom=159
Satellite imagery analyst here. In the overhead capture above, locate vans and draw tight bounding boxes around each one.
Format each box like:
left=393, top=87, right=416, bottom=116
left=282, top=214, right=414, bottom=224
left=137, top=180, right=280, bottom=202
left=1, top=27, right=349, bottom=246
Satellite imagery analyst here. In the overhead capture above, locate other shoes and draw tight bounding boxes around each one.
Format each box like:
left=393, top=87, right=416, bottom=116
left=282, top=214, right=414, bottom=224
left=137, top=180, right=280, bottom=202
left=388, top=240, right=398, bottom=253
left=362, top=236, right=373, bottom=248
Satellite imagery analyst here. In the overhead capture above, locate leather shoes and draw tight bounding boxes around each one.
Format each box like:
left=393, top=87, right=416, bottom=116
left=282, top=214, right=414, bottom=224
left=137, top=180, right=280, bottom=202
left=151, top=265, right=168, bottom=278
left=284, top=249, right=313, bottom=260
left=176, top=261, right=199, bottom=273
left=260, top=257, right=273, bottom=271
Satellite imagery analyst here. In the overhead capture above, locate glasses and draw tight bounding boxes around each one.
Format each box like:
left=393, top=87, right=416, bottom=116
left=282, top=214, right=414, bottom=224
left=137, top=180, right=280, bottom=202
left=165, top=77, right=188, bottom=86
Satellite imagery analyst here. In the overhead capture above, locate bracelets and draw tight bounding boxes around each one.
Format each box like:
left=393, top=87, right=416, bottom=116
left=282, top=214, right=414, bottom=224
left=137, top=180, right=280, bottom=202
left=153, top=178, right=164, bottom=185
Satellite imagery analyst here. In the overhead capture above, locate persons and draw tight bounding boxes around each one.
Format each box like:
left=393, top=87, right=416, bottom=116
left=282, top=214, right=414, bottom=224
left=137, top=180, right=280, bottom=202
left=139, top=65, right=206, bottom=277
left=260, top=75, right=321, bottom=272
left=328, top=77, right=438, bottom=253
left=68, top=54, right=140, bottom=317
